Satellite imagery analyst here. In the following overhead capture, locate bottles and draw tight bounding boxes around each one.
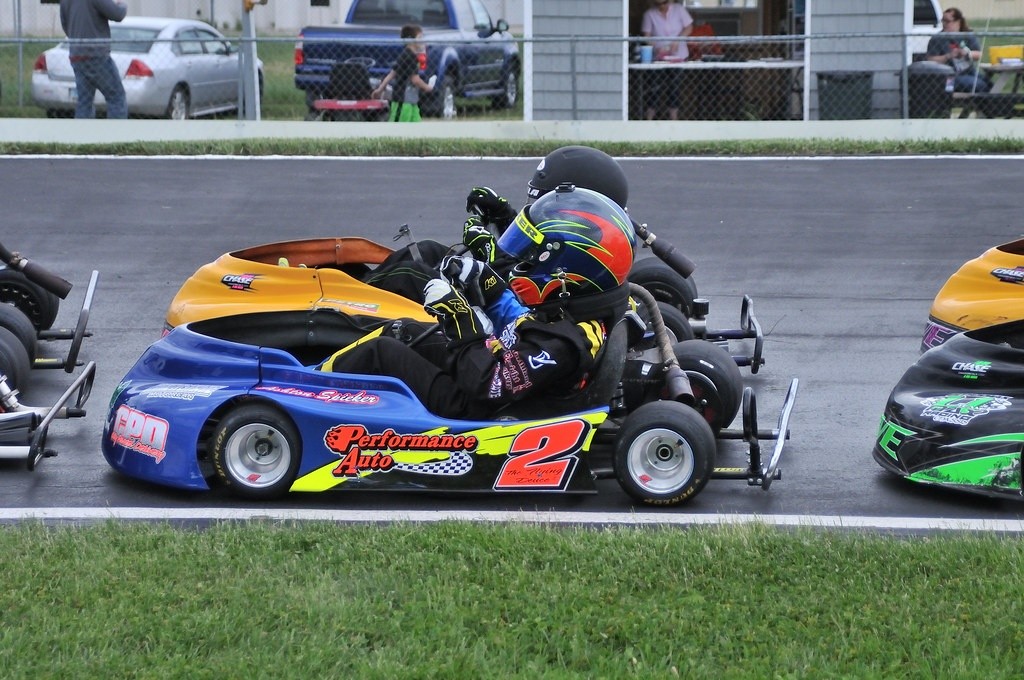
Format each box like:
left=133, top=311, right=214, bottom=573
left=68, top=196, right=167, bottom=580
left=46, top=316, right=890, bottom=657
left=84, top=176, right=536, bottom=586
left=427, top=71, right=438, bottom=92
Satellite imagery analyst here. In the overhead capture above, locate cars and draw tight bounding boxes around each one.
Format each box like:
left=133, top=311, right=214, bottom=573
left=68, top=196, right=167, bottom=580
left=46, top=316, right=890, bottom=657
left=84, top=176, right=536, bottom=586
left=32, top=15, right=266, bottom=119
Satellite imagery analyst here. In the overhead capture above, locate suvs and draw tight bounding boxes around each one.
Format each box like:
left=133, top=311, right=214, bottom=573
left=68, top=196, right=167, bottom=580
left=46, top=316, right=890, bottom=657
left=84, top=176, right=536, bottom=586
left=912, top=0, right=943, bottom=62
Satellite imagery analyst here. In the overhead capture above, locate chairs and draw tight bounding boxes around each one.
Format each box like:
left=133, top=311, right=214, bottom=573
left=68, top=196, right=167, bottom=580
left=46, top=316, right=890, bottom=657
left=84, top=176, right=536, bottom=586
left=424, top=9, right=441, bottom=26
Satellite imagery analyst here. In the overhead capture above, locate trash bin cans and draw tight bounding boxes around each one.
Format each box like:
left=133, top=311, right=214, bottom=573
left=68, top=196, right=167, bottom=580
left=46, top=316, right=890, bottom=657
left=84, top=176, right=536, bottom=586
left=895, top=61, right=955, bottom=119
left=816, top=71, right=875, bottom=120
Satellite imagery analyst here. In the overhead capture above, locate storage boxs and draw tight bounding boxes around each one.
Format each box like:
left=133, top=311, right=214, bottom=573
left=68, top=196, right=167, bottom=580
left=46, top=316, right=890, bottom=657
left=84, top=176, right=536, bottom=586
left=988, top=45, right=1024, bottom=65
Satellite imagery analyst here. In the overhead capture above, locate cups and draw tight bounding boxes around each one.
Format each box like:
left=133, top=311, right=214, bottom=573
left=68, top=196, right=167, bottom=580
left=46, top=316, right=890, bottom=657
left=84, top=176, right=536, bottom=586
left=640, top=46, right=653, bottom=63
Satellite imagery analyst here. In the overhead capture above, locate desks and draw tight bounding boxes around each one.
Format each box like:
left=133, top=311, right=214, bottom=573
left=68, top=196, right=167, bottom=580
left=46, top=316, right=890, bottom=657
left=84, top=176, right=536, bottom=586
left=629, top=58, right=805, bottom=121
left=979, top=63, right=1024, bottom=94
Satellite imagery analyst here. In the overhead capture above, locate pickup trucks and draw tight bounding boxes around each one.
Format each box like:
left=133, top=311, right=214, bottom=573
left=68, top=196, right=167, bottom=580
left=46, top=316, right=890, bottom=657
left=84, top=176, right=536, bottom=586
left=293, top=0, right=523, bottom=121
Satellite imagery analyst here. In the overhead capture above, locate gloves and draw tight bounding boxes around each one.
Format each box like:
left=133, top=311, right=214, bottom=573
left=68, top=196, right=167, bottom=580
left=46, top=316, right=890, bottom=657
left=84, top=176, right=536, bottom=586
left=440, top=254, right=509, bottom=308
left=422, top=279, right=493, bottom=352
left=465, top=186, right=518, bottom=236
left=462, top=217, right=508, bottom=263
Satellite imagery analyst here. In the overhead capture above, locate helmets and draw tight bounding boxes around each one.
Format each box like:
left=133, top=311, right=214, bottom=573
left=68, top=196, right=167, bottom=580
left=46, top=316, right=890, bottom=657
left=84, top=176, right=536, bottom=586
left=496, top=182, right=638, bottom=319
left=525, top=146, right=629, bottom=213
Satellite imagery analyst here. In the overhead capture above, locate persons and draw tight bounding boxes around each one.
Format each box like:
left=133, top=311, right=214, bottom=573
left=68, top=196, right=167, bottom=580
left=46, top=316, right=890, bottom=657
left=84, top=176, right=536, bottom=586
left=642, top=0, right=694, bottom=119
left=308, top=144, right=636, bottom=422
left=927, top=9, right=989, bottom=116
left=59, top=0, right=127, bottom=118
left=372, top=24, right=433, bottom=122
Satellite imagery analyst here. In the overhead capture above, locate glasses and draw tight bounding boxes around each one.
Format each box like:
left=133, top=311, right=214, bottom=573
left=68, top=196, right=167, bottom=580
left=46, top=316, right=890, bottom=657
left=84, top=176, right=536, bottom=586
left=655, top=0, right=668, bottom=6
left=941, top=18, right=952, bottom=24
